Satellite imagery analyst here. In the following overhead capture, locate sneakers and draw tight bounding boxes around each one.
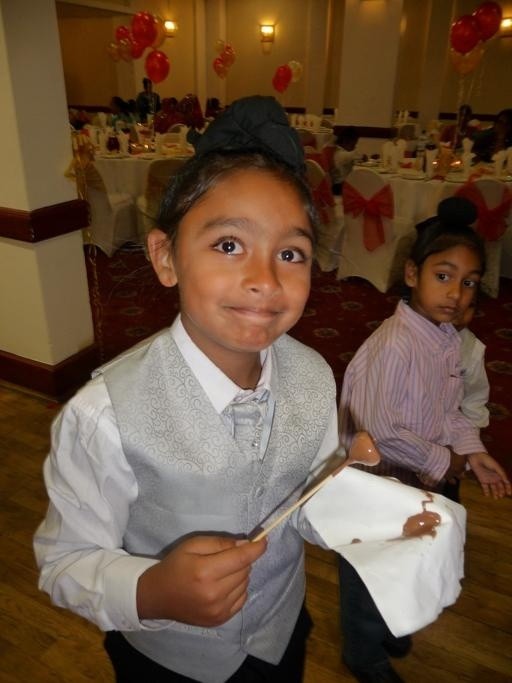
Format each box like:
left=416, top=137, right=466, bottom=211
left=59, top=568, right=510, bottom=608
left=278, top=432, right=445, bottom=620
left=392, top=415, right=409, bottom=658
left=343, top=639, right=403, bottom=682
left=386, top=632, right=411, bottom=657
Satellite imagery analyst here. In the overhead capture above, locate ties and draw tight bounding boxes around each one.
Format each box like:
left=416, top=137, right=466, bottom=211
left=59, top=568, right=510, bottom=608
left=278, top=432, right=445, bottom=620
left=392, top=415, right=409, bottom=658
left=233, top=400, right=268, bottom=455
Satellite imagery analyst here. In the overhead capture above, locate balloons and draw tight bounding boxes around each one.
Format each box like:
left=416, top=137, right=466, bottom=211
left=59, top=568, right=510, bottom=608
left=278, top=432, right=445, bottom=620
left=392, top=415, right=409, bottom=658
left=271, top=60, right=302, bottom=93
left=448, top=0, right=502, bottom=73
left=213, top=39, right=237, bottom=82
left=107, top=12, right=170, bottom=83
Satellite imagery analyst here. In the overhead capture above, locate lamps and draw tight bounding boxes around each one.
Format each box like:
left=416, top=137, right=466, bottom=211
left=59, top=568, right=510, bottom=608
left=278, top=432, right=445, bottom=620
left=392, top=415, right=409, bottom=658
left=162, top=17, right=179, bottom=38
left=258, top=21, right=276, bottom=51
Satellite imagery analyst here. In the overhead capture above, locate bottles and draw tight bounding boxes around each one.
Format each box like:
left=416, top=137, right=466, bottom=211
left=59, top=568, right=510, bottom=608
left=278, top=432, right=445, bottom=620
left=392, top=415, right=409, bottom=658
left=414, top=128, right=440, bottom=175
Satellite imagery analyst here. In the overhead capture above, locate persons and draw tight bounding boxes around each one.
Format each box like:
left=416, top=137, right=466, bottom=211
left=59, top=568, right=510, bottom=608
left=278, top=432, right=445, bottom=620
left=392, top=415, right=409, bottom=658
left=439, top=104, right=476, bottom=148
left=339, top=226, right=510, bottom=683
left=136, top=77, right=160, bottom=114
left=472, top=109, right=511, bottom=160
left=30, top=141, right=398, bottom=682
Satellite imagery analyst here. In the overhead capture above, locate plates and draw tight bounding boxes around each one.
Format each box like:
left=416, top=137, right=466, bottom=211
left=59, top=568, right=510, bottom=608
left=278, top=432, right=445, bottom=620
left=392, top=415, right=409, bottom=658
left=355, top=156, right=512, bottom=187
left=102, top=149, right=196, bottom=159
